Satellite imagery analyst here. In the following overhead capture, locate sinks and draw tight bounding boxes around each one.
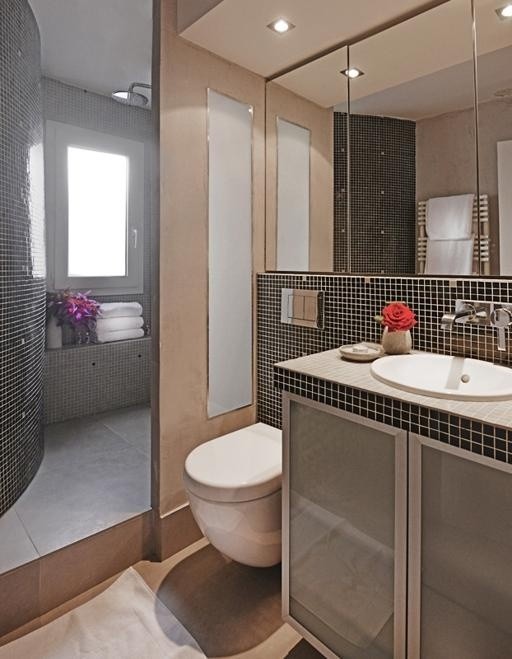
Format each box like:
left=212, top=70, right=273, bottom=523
left=370, top=353, right=512, bottom=401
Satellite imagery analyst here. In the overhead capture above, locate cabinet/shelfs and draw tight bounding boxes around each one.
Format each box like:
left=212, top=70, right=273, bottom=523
left=280, top=390, right=511, bottom=659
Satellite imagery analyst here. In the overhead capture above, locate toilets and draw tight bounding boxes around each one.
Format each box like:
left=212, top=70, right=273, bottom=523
left=182, top=421, right=282, bottom=568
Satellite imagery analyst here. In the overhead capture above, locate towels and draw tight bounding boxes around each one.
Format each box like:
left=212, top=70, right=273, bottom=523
left=90, top=301, right=144, bottom=343
left=424, top=193, right=475, bottom=276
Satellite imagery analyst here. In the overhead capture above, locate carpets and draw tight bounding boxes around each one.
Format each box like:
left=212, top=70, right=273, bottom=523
left=0, top=566, right=208, bottom=659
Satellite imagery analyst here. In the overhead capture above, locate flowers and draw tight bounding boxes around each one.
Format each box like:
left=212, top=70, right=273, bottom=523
left=372, top=304, right=418, bottom=331
left=44, top=287, right=102, bottom=321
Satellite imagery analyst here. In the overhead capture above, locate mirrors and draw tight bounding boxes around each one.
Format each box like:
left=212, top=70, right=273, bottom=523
left=264, top=1, right=511, bottom=279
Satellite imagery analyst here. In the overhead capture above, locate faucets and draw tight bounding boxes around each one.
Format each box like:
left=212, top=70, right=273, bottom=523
left=441, top=308, right=486, bottom=331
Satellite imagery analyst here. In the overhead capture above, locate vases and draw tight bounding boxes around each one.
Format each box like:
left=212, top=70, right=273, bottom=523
left=46, top=312, right=63, bottom=349
left=382, top=325, right=412, bottom=354
